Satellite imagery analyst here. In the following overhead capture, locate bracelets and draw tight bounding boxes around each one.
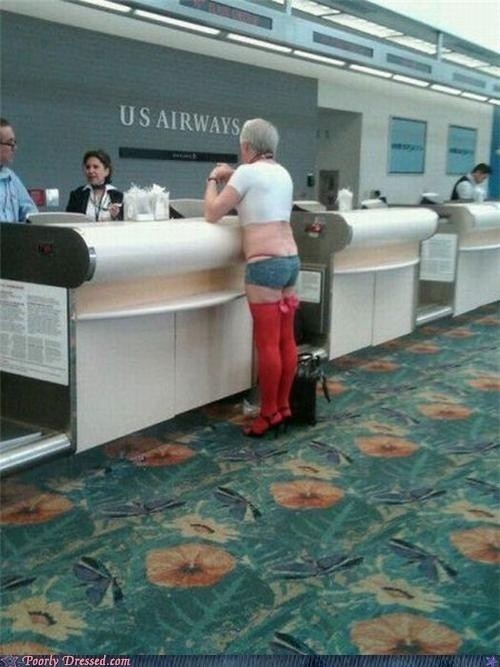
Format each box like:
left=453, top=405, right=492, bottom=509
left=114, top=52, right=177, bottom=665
left=208, top=177, right=219, bottom=184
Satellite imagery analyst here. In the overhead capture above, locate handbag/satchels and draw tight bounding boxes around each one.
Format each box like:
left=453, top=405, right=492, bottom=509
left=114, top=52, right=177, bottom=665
left=292, top=352, right=330, bottom=426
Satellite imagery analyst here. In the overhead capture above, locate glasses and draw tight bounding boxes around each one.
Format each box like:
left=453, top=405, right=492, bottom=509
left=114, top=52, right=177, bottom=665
left=0, top=140, right=17, bottom=150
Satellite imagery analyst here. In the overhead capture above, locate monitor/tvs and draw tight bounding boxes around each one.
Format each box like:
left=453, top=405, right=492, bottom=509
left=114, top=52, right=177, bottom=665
left=419, top=192, right=445, bottom=204
left=360, top=198, right=387, bottom=209
left=169, top=198, right=204, bottom=218
left=293, top=200, right=327, bottom=212
left=24, top=211, right=92, bottom=225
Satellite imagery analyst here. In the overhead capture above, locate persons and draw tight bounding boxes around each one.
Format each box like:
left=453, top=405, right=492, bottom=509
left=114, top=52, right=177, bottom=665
left=450, top=162, right=493, bottom=202
left=0, top=116, right=40, bottom=223
left=204, top=118, right=302, bottom=439
left=65, top=148, right=123, bottom=222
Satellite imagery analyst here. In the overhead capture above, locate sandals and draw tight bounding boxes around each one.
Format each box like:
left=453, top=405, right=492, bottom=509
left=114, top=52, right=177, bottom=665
left=242, top=406, right=292, bottom=439
